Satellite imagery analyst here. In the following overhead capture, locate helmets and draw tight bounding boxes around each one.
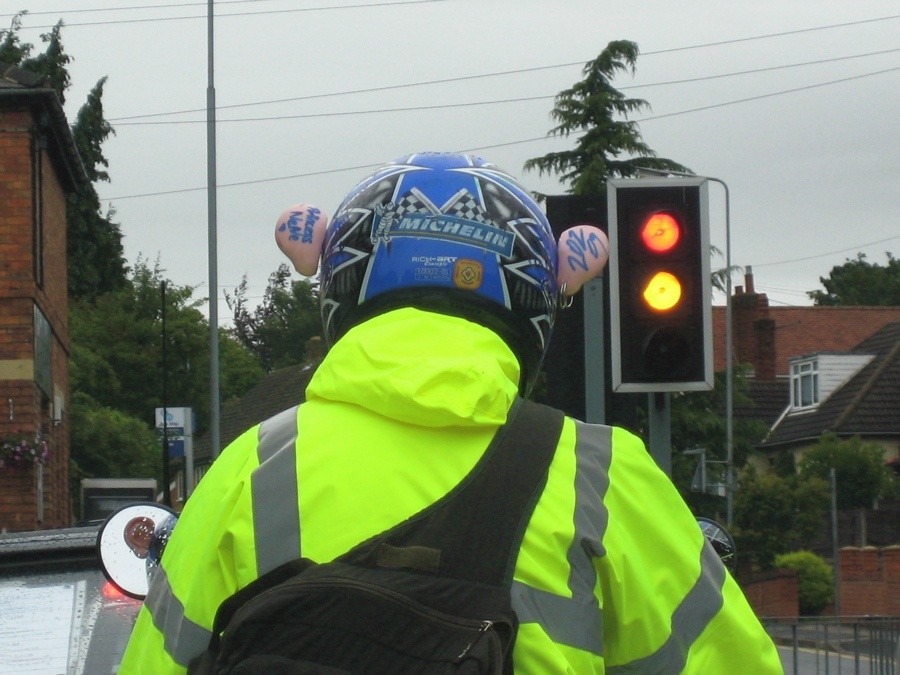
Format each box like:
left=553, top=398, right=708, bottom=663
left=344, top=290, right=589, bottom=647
left=316, top=148, right=557, bottom=399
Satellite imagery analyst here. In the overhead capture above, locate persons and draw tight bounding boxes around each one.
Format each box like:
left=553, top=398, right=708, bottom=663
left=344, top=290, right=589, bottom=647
left=115, top=150, right=784, bottom=675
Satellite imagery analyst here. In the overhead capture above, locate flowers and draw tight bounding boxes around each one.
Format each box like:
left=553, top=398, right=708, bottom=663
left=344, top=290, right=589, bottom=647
left=0, top=425, right=52, bottom=476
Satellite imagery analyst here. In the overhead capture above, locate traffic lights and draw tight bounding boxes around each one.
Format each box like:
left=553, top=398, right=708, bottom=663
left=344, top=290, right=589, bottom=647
left=607, top=177, right=714, bottom=393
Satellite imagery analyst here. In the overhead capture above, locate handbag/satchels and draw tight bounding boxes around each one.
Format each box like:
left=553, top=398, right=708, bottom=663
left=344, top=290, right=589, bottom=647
left=190, top=549, right=518, bottom=675
